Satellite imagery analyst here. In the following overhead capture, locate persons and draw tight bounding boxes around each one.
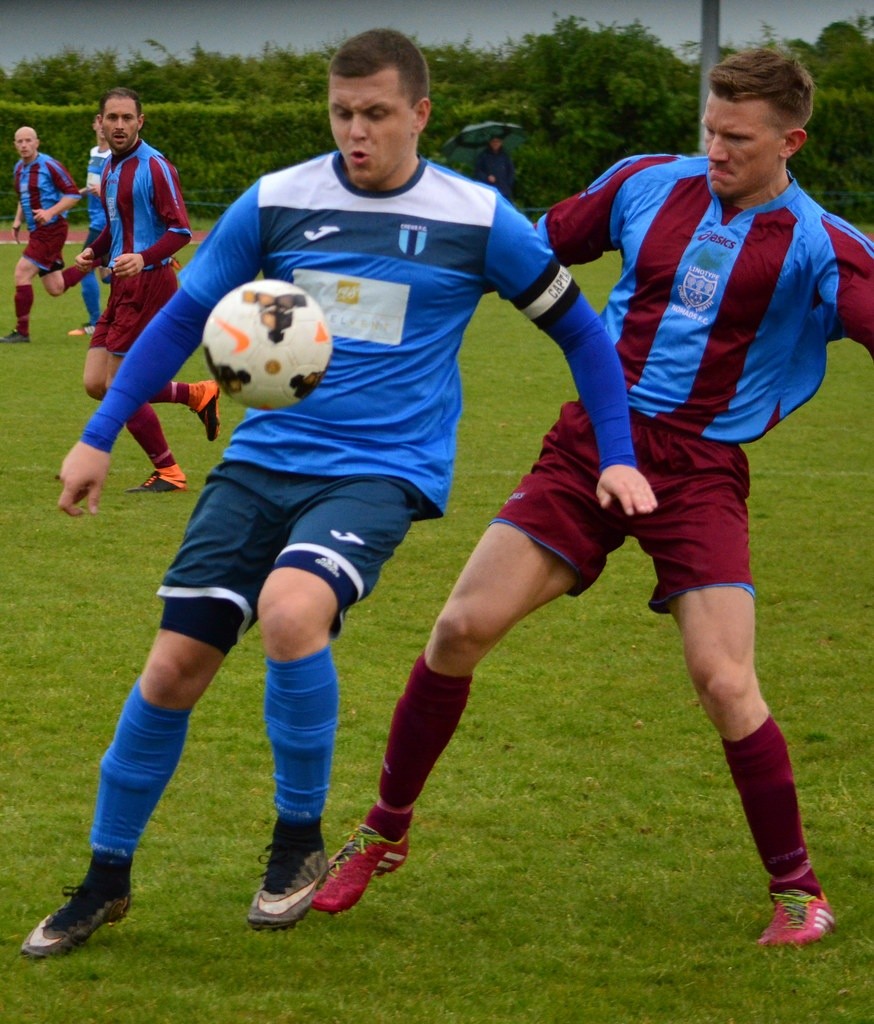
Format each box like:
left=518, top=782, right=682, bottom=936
left=314, top=54, right=873, bottom=951
left=21, top=28, right=663, bottom=961
left=68, top=113, right=114, bottom=337
left=75, top=87, right=220, bottom=493
left=0, top=126, right=111, bottom=345
left=476, top=135, right=514, bottom=202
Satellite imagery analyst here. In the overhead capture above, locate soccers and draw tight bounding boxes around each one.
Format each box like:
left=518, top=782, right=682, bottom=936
left=203, top=279, right=334, bottom=411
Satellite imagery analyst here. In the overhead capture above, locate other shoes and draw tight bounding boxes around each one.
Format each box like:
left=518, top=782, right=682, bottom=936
left=169, top=258, right=181, bottom=270
left=68, top=325, right=95, bottom=336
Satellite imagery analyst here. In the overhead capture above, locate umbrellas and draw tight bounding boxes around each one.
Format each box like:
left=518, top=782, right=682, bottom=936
left=436, top=120, right=523, bottom=166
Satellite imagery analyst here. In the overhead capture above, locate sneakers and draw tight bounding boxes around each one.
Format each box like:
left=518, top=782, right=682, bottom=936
left=759, top=890, right=834, bottom=947
left=189, top=380, right=220, bottom=441
left=311, top=820, right=409, bottom=912
left=124, top=472, right=187, bottom=492
left=20, top=883, right=132, bottom=956
left=0, top=329, right=30, bottom=343
left=246, top=845, right=330, bottom=927
